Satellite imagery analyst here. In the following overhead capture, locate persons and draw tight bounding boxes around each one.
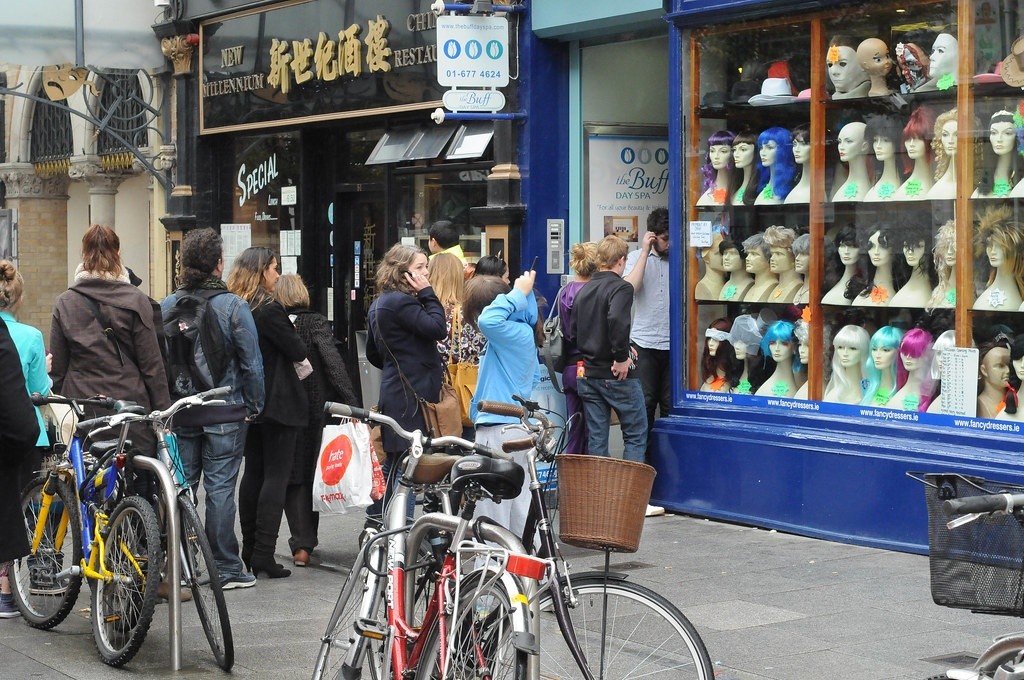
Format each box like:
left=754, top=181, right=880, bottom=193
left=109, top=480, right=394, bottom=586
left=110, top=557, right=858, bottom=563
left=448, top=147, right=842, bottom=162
left=972, top=205, right=1023, bottom=311
left=826, top=29, right=982, bottom=98
left=971, top=101, right=1024, bottom=198
left=276, top=274, right=361, bottom=566
left=558, top=236, right=666, bottom=516
left=831, top=103, right=985, bottom=201
left=824, top=325, right=975, bottom=415
left=977, top=326, right=1024, bottom=423
left=695, top=224, right=834, bottom=304
left=0, top=316, right=40, bottom=564
left=701, top=311, right=830, bottom=402
left=162, top=230, right=265, bottom=590
left=0, top=259, right=53, bottom=619
left=226, top=247, right=309, bottom=579
left=623, top=209, right=672, bottom=431
left=696, top=123, right=828, bottom=205
left=820, top=222, right=979, bottom=308
left=50, top=224, right=194, bottom=602
left=364, top=221, right=547, bottom=569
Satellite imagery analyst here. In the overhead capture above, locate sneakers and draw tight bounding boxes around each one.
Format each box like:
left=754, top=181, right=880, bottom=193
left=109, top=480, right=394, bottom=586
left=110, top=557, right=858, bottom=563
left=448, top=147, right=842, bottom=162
left=221, top=572, right=257, bottom=590
left=27, top=575, right=70, bottom=596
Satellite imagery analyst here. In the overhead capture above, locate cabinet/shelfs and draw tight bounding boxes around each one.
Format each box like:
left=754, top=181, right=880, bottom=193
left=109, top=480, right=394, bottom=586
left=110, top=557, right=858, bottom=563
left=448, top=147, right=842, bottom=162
left=694, top=81, right=1024, bottom=322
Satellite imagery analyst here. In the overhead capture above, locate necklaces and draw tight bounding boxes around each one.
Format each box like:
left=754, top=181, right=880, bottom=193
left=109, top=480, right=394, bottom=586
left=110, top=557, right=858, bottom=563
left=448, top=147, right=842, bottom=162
left=793, top=285, right=809, bottom=305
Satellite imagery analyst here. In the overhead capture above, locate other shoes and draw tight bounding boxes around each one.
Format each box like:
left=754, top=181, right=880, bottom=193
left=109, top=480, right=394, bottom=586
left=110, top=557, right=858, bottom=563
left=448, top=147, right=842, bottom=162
left=152, top=581, right=192, bottom=604
left=292, top=548, right=309, bottom=567
left=0, top=592, right=22, bottom=619
left=644, top=503, right=666, bottom=517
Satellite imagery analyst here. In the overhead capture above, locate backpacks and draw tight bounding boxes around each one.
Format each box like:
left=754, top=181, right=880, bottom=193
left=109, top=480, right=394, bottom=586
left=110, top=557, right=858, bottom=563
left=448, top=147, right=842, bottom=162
left=157, top=286, right=238, bottom=402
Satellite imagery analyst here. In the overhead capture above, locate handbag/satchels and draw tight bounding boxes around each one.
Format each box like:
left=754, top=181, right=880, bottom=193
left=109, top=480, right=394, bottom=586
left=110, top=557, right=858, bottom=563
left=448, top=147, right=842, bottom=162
left=539, top=288, right=564, bottom=393
left=311, top=416, right=387, bottom=517
left=445, top=359, right=485, bottom=426
left=413, top=379, right=465, bottom=447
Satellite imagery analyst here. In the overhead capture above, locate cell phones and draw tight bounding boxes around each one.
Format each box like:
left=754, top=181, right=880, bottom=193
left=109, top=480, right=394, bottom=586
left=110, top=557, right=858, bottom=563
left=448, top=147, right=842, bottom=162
left=401, top=270, right=413, bottom=284
left=530, top=256, right=539, bottom=273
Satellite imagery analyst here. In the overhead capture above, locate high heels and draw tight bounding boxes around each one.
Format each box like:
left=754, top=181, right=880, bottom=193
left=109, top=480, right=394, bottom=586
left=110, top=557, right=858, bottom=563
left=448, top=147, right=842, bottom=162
left=250, top=551, right=292, bottom=579
left=242, top=556, right=284, bottom=573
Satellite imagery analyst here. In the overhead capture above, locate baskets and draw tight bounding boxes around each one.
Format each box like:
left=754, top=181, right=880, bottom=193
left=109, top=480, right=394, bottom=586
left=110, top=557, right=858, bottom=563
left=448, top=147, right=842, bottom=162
left=904, top=470, right=1024, bottom=619
left=552, top=452, right=658, bottom=553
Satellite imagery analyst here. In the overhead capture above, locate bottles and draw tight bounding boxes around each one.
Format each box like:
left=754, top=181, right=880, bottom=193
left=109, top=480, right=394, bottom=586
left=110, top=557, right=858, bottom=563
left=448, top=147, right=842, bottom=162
left=576, top=361, right=585, bottom=378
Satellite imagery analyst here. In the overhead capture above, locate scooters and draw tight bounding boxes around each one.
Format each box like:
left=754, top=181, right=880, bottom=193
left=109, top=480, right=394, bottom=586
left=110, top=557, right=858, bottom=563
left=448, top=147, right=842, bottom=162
left=905, top=467, right=1024, bottom=680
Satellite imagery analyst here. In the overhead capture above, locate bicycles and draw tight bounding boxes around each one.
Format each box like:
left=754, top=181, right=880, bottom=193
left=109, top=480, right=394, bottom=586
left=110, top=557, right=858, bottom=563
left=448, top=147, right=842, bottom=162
left=6, top=391, right=163, bottom=668
left=359, top=394, right=715, bottom=680
left=312, top=399, right=551, bottom=680
left=74, top=385, right=235, bottom=671
